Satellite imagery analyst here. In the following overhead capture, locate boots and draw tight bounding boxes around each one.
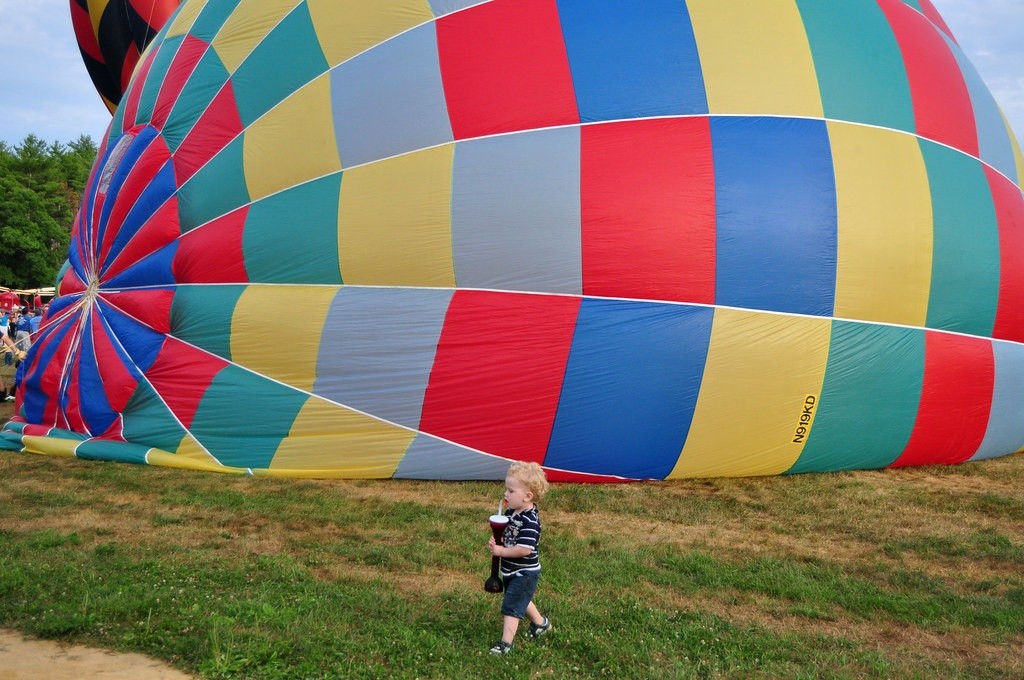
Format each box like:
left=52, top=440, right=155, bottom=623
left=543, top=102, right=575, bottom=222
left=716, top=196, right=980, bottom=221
left=0, top=387, right=9, bottom=403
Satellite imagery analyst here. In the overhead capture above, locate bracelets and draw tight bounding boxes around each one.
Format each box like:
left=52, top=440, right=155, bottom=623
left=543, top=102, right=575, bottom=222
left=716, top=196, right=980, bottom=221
left=14, top=349, right=21, bottom=356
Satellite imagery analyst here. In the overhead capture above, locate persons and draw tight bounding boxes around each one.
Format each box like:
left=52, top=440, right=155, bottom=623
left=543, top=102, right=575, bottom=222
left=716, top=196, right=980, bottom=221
left=488, top=461, right=553, bottom=657
left=0, top=307, right=43, bottom=406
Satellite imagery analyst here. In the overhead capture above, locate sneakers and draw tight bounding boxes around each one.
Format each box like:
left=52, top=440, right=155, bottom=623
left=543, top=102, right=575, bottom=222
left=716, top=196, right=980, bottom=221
left=488, top=640, right=513, bottom=656
left=523, top=616, right=552, bottom=639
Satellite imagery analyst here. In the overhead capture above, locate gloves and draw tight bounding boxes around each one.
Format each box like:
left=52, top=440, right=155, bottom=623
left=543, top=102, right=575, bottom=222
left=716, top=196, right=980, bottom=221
left=14, top=348, right=28, bottom=364
